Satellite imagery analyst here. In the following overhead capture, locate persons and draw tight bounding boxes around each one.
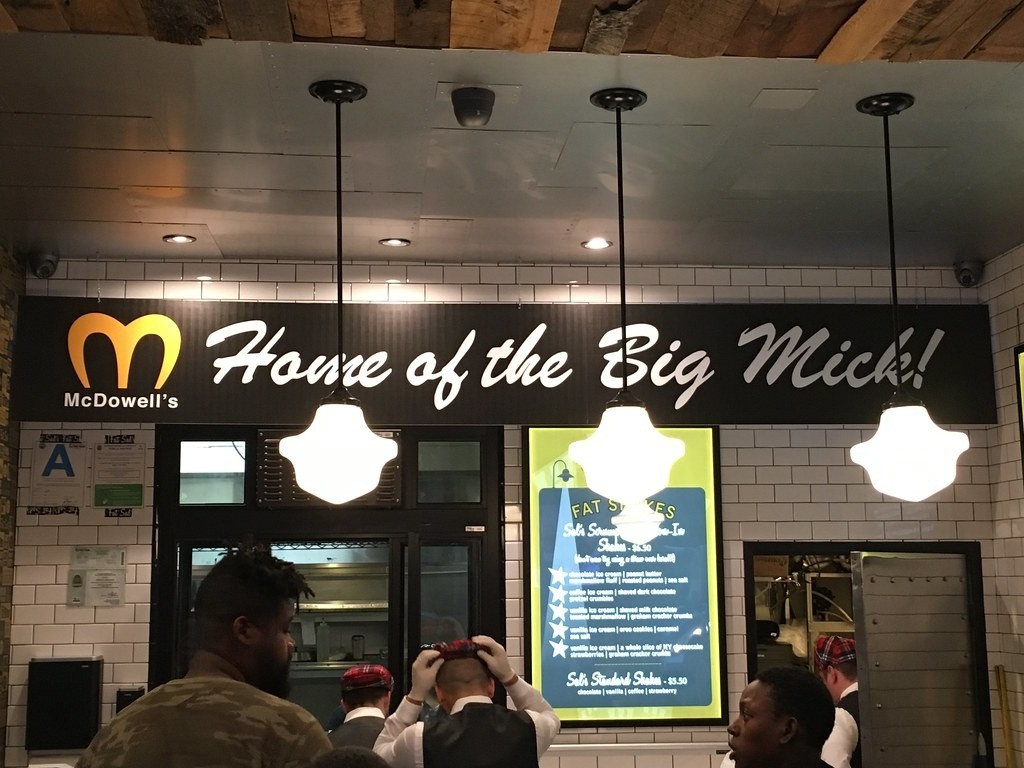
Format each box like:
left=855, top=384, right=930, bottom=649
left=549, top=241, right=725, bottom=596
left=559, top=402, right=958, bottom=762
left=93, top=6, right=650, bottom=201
left=309, top=747, right=389, bottom=768
left=726, top=666, right=837, bottom=768
left=325, top=663, right=395, bottom=748
left=75, top=535, right=336, bottom=768
left=813, top=633, right=864, bottom=768
left=372, top=636, right=562, bottom=767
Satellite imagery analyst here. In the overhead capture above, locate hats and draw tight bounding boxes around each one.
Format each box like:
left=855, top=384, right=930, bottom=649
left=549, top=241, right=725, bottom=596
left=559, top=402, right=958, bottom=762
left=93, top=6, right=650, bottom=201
left=341, top=664, right=395, bottom=691
left=429, top=639, right=492, bottom=664
left=813, top=634, right=858, bottom=671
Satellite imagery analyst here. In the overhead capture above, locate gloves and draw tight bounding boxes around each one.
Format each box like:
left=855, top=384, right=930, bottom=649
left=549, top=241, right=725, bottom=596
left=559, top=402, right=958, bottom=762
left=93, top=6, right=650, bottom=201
left=407, top=649, right=445, bottom=702
left=472, top=635, right=515, bottom=684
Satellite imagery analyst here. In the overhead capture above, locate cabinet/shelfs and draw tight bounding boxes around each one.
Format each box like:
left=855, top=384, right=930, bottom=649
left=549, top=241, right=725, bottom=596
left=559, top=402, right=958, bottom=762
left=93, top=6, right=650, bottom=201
left=753, top=572, right=855, bottom=671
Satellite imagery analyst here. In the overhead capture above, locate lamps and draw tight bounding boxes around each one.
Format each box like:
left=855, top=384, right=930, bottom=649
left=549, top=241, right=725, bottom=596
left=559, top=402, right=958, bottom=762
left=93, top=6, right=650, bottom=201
left=274, top=80, right=401, bottom=511
left=849, top=91, right=970, bottom=503
left=558, top=86, right=690, bottom=510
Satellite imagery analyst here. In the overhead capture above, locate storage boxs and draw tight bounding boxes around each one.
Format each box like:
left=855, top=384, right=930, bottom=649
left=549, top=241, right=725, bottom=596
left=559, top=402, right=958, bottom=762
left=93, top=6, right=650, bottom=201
left=753, top=554, right=789, bottom=578
